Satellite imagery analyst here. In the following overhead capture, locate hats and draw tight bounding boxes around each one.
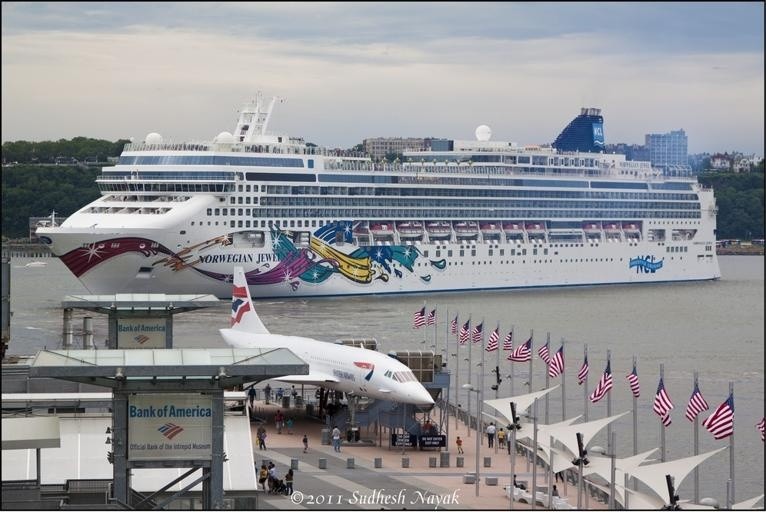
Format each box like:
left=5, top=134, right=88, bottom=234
left=715, top=238, right=764, bottom=250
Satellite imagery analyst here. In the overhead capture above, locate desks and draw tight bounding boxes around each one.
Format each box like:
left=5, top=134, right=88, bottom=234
left=515, top=397, right=537, bottom=510
left=459, top=380, right=481, bottom=498
left=695, top=496, right=719, bottom=510
left=585, top=444, right=616, bottom=511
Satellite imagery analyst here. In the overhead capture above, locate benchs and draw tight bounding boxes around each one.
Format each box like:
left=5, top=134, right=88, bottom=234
left=450, top=317, right=734, bottom=441
left=425, top=309, right=435, bottom=327
left=413, top=307, right=425, bottom=329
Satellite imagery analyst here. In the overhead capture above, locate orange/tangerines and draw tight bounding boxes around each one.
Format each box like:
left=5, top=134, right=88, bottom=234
left=268, top=477, right=286, bottom=495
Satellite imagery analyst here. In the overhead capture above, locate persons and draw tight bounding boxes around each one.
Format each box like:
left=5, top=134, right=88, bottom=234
left=456, top=436, right=464, bottom=455
left=486, top=422, right=495, bottom=448
left=555, top=472, right=564, bottom=483
left=303, top=435, right=308, bottom=452
left=422, top=421, right=432, bottom=434
left=505, top=430, right=511, bottom=455
left=254, top=461, right=293, bottom=495
left=514, top=475, right=519, bottom=488
left=248, top=384, right=297, bottom=450
left=520, top=484, right=526, bottom=489
left=552, top=485, right=559, bottom=496
left=498, top=428, right=505, bottom=449
left=331, top=425, right=340, bottom=452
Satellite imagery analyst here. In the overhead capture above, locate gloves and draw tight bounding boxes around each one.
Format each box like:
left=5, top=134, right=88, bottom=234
left=214, top=261, right=437, bottom=445
left=26, top=260, right=47, bottom=267
